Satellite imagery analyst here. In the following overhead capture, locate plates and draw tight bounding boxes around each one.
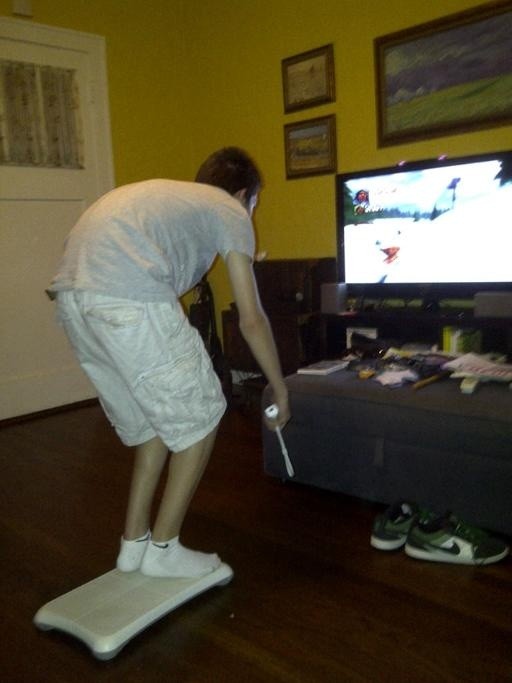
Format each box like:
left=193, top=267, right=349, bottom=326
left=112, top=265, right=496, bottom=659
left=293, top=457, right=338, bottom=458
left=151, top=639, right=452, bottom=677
left=317, top=257, right=511, bottom=377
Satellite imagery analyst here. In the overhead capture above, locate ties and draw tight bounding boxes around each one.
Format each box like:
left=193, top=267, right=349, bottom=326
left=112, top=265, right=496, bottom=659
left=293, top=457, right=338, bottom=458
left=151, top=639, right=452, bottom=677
left=333, top=148, right=512, bottom=326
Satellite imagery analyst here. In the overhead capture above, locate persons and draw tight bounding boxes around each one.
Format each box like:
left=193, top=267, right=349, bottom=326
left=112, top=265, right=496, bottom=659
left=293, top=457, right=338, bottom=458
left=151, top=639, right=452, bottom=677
left=45, top=146, right=291, bottom=576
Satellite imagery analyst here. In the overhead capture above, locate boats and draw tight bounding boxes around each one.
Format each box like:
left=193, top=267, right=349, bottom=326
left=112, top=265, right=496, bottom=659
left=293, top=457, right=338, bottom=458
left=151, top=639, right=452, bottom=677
left=319, top=282, right=347, bottom=314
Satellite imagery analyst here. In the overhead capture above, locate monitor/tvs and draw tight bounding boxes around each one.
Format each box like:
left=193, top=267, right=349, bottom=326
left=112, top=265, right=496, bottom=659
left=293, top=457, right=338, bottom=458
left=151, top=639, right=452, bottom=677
left=296, top=358, right=349, bottom=378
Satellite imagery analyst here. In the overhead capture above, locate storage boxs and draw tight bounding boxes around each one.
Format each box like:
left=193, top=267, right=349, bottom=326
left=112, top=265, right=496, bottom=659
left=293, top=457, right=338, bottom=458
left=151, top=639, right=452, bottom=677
left=261, top=341, right=512, bottom=540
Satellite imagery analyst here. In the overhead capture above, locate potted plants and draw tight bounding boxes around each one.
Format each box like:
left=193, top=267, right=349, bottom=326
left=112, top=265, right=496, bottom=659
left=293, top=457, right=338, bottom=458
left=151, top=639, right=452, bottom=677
left=282, top=113, right=337, bottom=180
left=372, top=0, right=512, bottom=150
left=281, top=42, right=336, bottom=114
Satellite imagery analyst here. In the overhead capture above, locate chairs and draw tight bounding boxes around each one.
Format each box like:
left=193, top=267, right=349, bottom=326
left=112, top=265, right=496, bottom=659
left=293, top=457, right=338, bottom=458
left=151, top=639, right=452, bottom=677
left=366, top=492, right=431, bottom=555
left=405, top=508, right=510, bottom=570
